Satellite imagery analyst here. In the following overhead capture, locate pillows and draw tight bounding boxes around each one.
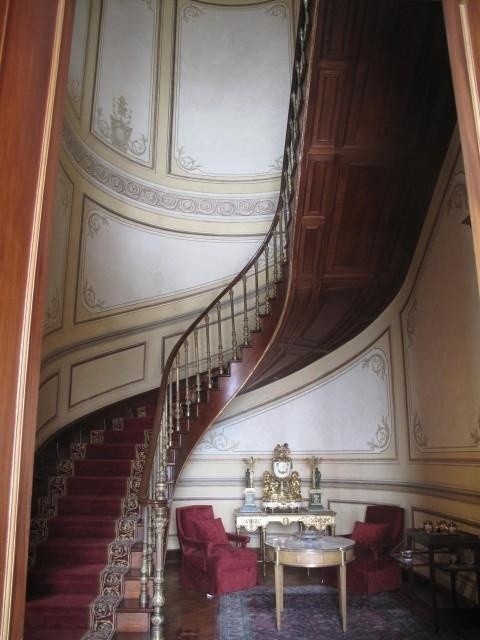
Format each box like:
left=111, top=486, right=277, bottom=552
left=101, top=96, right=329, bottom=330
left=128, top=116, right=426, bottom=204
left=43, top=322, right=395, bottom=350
left=351, top=509, right=395, bottom=549
left=180, top=508, right=230, bottom=548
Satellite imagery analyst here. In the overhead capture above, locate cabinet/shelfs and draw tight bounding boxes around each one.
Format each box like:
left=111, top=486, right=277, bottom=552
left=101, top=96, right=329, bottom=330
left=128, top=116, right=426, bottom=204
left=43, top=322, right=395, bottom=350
left=404, top=528, right=480, bottom=634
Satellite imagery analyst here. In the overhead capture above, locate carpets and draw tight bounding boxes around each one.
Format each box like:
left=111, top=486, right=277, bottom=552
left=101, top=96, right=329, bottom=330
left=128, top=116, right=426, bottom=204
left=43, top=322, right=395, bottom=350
left=218, top=586, right=465, bottom=640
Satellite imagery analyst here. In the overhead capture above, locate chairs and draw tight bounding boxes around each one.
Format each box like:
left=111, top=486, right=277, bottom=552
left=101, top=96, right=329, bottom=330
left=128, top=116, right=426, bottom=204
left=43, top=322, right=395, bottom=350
left=321, top=505, right=405, bottom=594
left=175, top=505, right=258, bottom=596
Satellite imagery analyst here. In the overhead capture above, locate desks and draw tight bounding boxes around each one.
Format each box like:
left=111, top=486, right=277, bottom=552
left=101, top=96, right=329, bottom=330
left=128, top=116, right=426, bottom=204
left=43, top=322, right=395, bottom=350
left=233, top=509, right=337, bottom=568
